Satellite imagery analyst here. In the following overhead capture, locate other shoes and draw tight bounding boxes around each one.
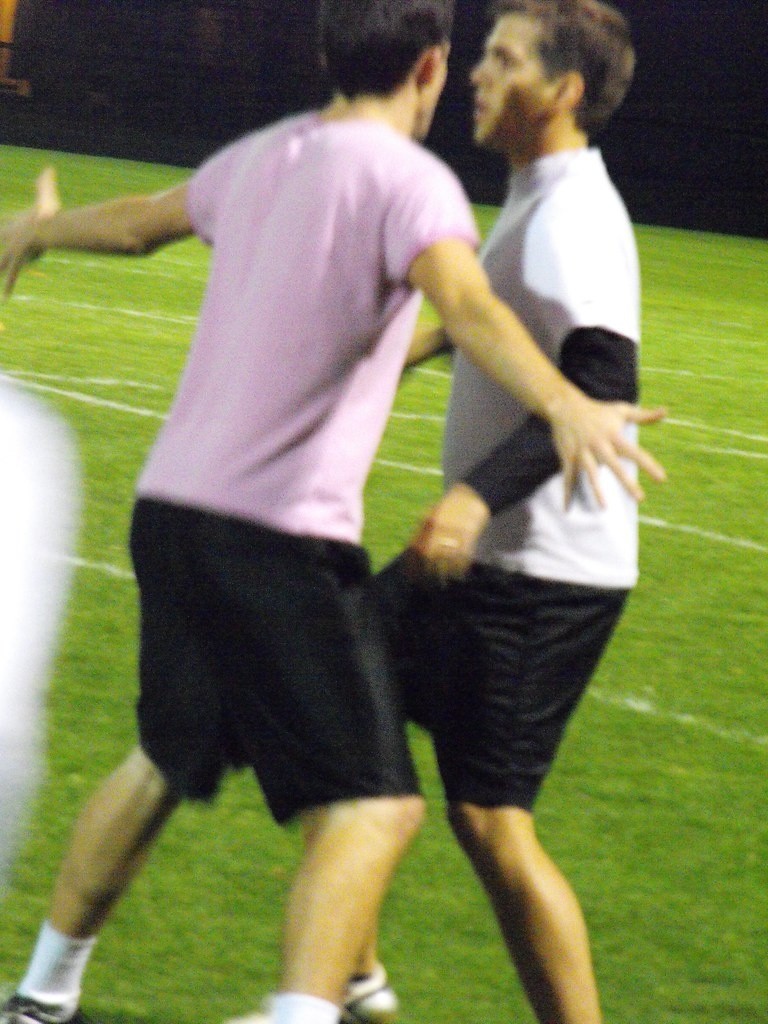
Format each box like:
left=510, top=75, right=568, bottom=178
left=343, top=964, right=401, bottom=1021
left=1, top=988, right=94, bottom=1024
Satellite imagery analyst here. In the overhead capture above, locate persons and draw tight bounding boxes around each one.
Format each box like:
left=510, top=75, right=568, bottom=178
left=342, top=2, right=642, bottom=1023
left=0, top=1, right=670, bottom=1023
